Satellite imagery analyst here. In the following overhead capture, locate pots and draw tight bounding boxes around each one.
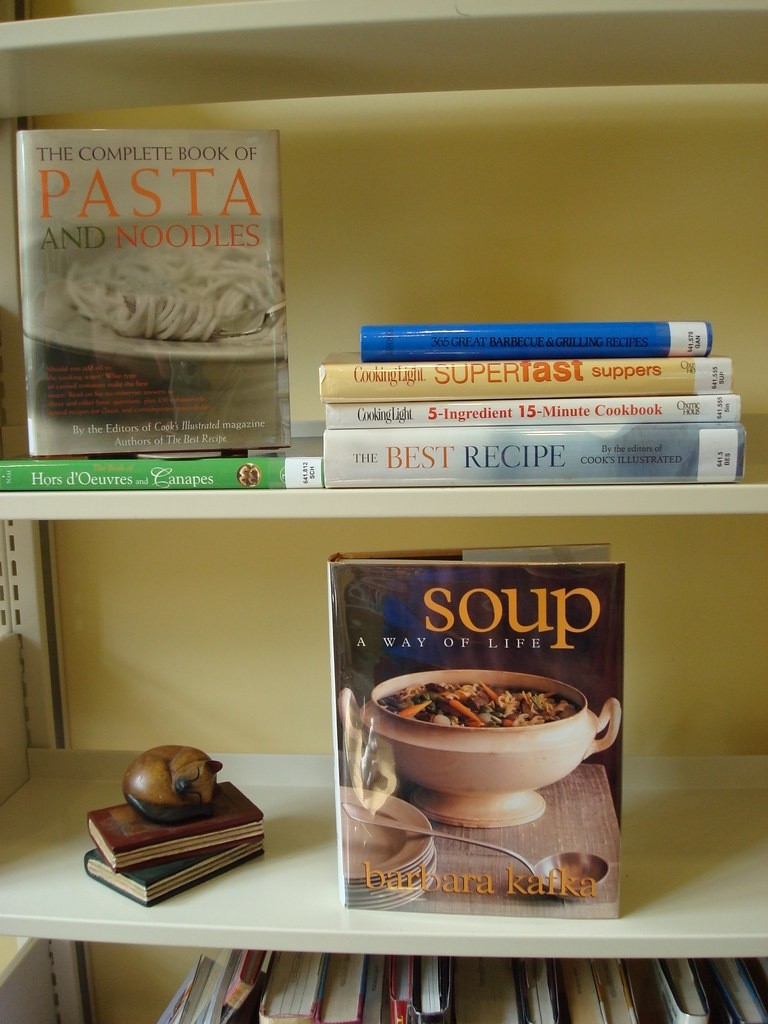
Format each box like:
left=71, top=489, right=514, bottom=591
left=338, top=669, right=621, bottom=828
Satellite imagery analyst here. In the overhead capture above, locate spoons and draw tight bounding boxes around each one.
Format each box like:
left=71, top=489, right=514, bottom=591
left=341, top=802, right=610, bottom=901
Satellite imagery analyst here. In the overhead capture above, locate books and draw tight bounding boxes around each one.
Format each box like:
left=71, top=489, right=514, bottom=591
left=85, top=781, right=265, bottom=907
left=155, top=949, right=768, bottom=1024
left=0, top=128, right=748, bottom=490
left=327, top=543, right=626, bottom=920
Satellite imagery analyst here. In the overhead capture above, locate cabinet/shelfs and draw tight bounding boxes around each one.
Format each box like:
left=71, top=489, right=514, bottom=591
left=0, top=0, right=768, bottom=1024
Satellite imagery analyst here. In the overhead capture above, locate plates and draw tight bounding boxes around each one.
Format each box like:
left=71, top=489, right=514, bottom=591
left=340, top=785, right=438, bottom=911
left=24, top=304, right=288, bottom=390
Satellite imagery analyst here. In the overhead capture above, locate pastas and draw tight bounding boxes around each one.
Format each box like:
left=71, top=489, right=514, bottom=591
left=380, top=681, right=578, bottom=728
left=64, top=242, right=285, bottom=345
